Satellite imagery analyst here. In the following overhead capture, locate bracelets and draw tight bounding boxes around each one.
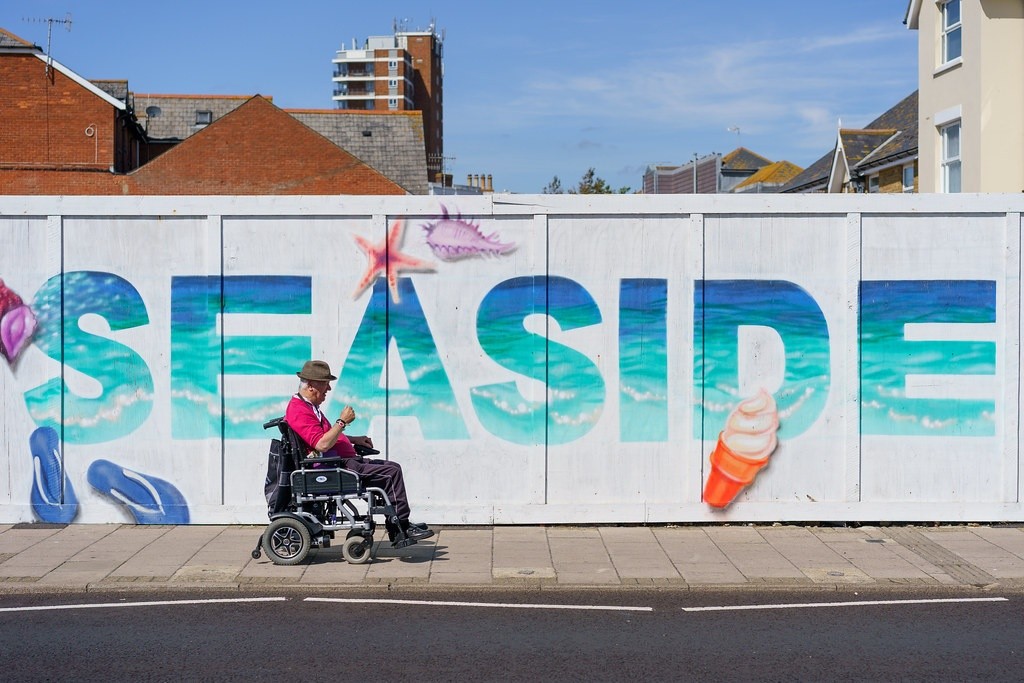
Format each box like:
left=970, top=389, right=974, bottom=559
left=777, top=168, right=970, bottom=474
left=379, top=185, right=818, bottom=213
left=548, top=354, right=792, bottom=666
left=336, top=418, right=347, bottom=428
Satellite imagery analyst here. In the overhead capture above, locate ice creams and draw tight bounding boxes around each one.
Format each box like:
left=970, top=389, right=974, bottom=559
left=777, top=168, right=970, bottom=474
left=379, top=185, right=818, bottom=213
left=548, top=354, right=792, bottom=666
left=703, top=388, right=780, bottom=509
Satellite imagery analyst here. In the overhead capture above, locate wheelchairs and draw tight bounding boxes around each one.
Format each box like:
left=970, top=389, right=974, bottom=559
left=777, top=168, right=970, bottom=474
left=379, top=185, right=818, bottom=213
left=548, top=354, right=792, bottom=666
left=251, top=416, right=418, bottom=565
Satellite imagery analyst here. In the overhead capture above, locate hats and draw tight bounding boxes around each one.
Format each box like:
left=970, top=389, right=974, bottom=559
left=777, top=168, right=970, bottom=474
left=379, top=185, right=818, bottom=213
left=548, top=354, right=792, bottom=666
left=296, top=361, right=338, bottom=381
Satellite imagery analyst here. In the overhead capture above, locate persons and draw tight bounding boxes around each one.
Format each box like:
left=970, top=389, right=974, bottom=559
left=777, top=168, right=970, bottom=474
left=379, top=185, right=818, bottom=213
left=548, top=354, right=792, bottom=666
left=283, top=360, right=435, bottom=542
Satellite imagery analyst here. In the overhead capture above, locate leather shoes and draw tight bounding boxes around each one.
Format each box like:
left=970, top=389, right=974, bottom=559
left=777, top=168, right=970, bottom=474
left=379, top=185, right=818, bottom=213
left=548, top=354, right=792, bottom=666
left=389, top=524, right=434, bottom=543
left=409, top=521, right=428, bottom=530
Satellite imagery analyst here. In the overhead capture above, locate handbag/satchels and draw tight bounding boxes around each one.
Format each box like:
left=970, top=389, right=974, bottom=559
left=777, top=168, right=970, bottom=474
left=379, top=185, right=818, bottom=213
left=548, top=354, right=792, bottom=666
left=264, top=439, right=295, bottom=514
